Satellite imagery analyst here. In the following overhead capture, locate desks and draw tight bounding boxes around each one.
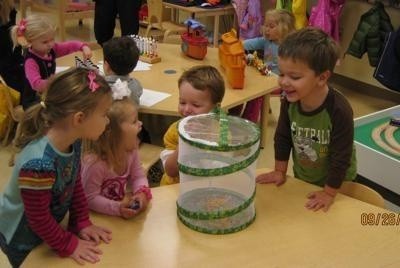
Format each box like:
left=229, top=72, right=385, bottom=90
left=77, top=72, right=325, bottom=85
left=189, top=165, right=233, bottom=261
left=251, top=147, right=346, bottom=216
left=162, top=1, right=240, bottom=47
left=56, top=43, right=282, bottom=150
left=19, top=167, right=399, bottom=267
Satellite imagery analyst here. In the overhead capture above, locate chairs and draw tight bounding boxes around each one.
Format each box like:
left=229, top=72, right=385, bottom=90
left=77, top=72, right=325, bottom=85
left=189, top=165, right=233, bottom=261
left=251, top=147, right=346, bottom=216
left=0, top=76, right=44, bottom=166
left=144, top=0, right=189, bottom=43
left=338, top=181, right=385, bottom=209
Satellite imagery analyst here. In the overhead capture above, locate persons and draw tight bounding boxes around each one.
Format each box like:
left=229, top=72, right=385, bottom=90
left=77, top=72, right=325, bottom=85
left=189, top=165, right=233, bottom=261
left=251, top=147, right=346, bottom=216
left=255, top=27, right=359, bottom=213
left=0, top=54, right=113, bottom=267
left=237, top=9, right=298, bottom=124
left=80, top=78, right=153, bottom=219
left=7, top=14, right=92, bottom=151
left=100, top=36, right=152, bottom=144
left=159, top=65, right=233, bottom=186
left=0, top=0, right=20, bottom=77
left=92, top=0, right=148, bottom=48
